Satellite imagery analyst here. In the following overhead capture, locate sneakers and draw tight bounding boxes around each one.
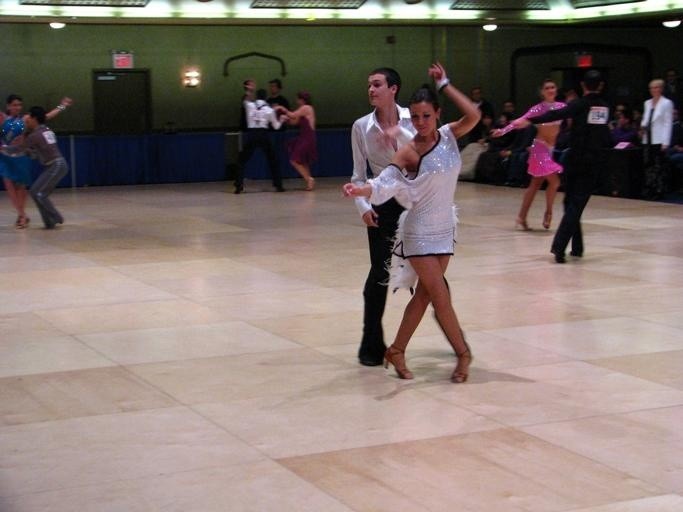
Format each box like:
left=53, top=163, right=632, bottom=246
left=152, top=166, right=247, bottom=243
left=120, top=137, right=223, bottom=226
left=358, top=338, right=387, bottom=368
left=568, top=250, right=583, bottom=258
left=232, top=182, right=245, bottom=195
left=270, top=179, right=287, bottom=193
left=550, top=247, right=567, bottom=264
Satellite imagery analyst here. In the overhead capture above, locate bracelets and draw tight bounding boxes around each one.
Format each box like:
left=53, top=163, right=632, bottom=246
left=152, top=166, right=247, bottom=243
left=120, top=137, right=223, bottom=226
left=436, top=78, right=450, bottom=95
left=56, top=103, right=66, bottom=111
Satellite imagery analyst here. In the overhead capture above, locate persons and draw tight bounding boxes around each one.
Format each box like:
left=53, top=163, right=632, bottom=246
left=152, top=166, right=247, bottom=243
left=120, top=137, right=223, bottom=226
left=266, top=79, right=289, bottom=117
left=342, top=62, right=483, bottom=384
left=240, top=80, right=257, bottom=131
left=272, top=91, right=317, bottom=191
left=234, top=89, right=290, bottom=194
left=350, top=68, right=417, bottom=366
left=492, top=70, right=611, bottom=262
left=469, top=75, right=683, bottom=200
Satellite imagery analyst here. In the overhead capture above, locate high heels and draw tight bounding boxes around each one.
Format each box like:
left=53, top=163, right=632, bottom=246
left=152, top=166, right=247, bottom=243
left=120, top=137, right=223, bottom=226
left=449, top=348, right=474, bottom=384
left=384, top=344, right=415, bottom=380
left=303, top=176, right=316, bottom=191
left=540, top=211, right=552, bottom=230
left=512, top=216, right=534, bottom=232
